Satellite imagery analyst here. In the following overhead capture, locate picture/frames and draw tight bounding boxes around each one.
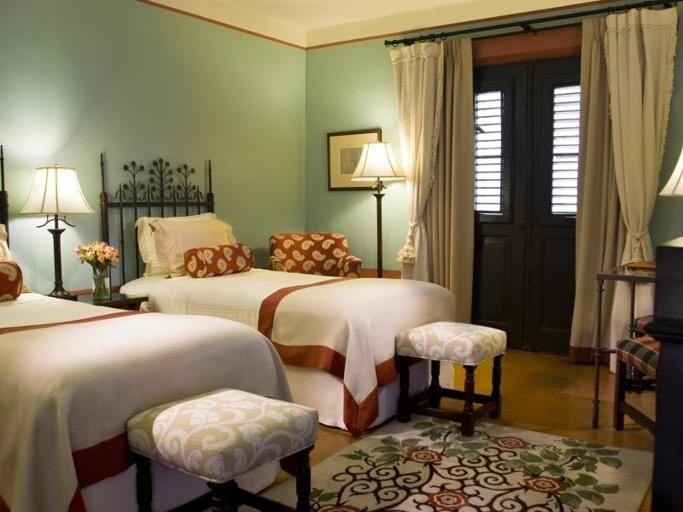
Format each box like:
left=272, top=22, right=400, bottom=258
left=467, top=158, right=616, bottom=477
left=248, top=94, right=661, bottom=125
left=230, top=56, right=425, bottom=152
left=326, top=127, right=382, bottom=192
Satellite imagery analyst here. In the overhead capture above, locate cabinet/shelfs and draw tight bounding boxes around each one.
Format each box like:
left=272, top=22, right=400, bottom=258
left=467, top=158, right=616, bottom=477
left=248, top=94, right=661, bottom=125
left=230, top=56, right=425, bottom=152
left=68, top=290, right=147, bottom=311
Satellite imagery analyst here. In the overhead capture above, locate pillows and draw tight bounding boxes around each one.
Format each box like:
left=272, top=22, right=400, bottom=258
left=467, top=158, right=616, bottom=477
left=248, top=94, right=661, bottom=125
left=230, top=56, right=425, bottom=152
left=0, top=260, right=22, bottom=301
left=146, top=217, right=235, bottom=281
left=132, top=211, right=217, bottom=278
left=184, top=242, right=252, bottom=278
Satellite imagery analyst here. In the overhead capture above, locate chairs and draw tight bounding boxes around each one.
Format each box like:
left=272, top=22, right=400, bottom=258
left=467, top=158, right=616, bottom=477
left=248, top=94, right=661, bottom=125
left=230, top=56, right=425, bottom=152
left=266, top=230, right=360, bottom=278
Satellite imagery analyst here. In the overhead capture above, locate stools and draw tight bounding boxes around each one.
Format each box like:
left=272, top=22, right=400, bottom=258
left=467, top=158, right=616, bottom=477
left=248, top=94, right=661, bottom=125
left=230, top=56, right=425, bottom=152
left=393, top=319, right=505, bottom=439
left=612, top=338, right=659, bottom=438
left=627, top=314, right=657, bottom=394
left=124, top=385, right=319, bottom=512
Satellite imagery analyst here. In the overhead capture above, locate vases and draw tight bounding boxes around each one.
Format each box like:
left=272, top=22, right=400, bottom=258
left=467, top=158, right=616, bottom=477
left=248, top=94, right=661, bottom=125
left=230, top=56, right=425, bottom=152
left=92, top=265, right=109, bottom=300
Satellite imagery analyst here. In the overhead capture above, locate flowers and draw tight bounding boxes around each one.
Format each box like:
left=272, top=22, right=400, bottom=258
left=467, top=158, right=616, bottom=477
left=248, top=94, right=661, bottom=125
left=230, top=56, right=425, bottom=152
left=73, top=238, right=117, bottom=268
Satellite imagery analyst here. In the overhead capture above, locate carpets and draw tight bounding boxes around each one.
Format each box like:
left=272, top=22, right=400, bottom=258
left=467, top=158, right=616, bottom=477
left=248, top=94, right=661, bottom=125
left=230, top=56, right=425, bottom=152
left=236, top=409, right=654, bottom=510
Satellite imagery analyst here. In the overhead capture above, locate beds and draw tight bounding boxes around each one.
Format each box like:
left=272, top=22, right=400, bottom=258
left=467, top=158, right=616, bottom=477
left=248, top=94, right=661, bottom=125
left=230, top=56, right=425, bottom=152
left=0, top=144, right=290, bottom=512
left=97, top=153, right=455, bottom=439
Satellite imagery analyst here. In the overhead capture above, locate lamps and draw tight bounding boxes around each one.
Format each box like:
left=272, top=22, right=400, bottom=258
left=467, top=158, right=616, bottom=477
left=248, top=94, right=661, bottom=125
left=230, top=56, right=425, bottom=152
left=654, top=136, right=683, bottom=200
left=350, top=137, right=404, bottom=276
left=17, top=164, right=94, bottom=300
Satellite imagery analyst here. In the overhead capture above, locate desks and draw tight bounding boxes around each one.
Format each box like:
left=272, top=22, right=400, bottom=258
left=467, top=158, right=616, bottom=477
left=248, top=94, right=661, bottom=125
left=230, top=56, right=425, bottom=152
left=651, top=235, right=681, bottom=512
left=590, top=258, right=656, bottom=432
